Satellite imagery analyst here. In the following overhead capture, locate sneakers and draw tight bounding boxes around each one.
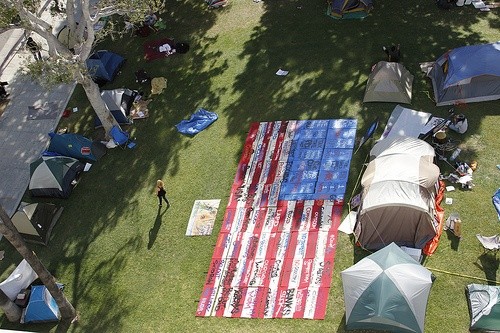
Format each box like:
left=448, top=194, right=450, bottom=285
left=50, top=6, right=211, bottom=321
left=167, top=204, right=171, bottom=206
left=159, top=205, right=162, bottom=206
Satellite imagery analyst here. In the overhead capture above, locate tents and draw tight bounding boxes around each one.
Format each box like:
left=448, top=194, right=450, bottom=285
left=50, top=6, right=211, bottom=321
left=0, top=17, right=142, bottom=323
left=326, top=0, right=373, bottom=20
left=340, top=105, right=500, bottom=333
left=427, top=41, right=500, bottom=106
left=204, top=0, right=229, bottom=10
left=363, top=61, right=414, bottom=104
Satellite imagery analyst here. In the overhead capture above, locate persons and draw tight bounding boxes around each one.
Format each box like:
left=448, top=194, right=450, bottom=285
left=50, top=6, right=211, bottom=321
left=25, top=37, right=43, bottom=63
left=449, top=113, right=468, bottom=134
left=156, top=180, right=171, bottom=207
left=134, top=68, right=147, bottom=83
left=145, top=11, right=159, bottom=32
left=383, top=43, right=400, bottom=63
